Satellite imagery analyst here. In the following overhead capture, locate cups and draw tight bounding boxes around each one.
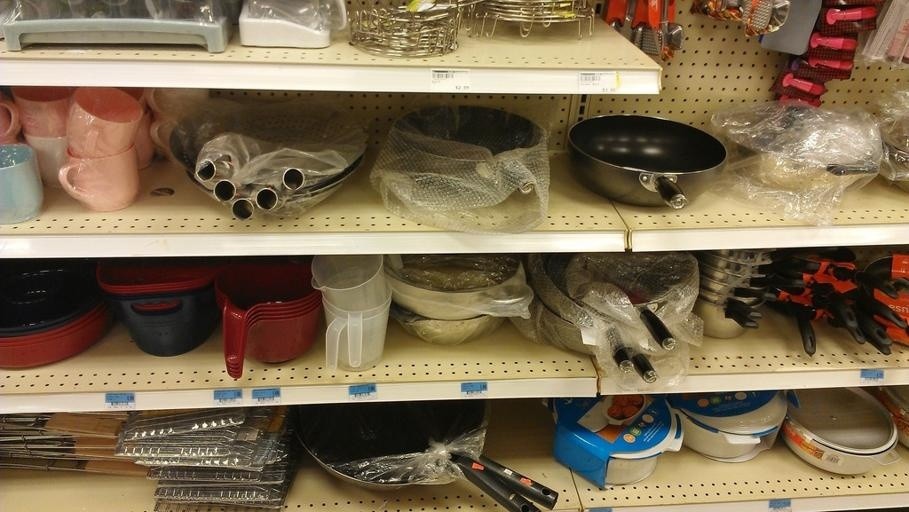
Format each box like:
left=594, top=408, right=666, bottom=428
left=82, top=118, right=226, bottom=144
left=307, top=252, right=405, bottom=377
left=0, top=78, right=207, bottom=228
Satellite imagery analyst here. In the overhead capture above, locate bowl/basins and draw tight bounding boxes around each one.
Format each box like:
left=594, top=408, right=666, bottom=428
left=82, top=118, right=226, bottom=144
left=0, top=259, right=116, bottom=371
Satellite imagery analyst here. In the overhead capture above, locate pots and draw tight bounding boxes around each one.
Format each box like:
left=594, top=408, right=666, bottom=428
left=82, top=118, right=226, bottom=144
left=866, top=112, right=909, bottom=197
left=379, top=252, right=537, bottom=322
left=384, top=300, right=512, bottom=344
left=286, top=398, right=559, bottom=512
left=561, top=114, right=729, bottom=213
left=878, top=385, right=909, bottom=453
left=524, top=247, right=705, bottom=386
left=663, top=237, right=909, bottom=357
left=389, top=105, right=546, bottom=199
left=717, top=104, right=882, bottom=201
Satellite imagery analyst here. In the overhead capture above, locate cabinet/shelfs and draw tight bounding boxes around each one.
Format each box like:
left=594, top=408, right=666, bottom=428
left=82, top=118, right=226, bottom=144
left=1, top=1, right=909, bottom=512
